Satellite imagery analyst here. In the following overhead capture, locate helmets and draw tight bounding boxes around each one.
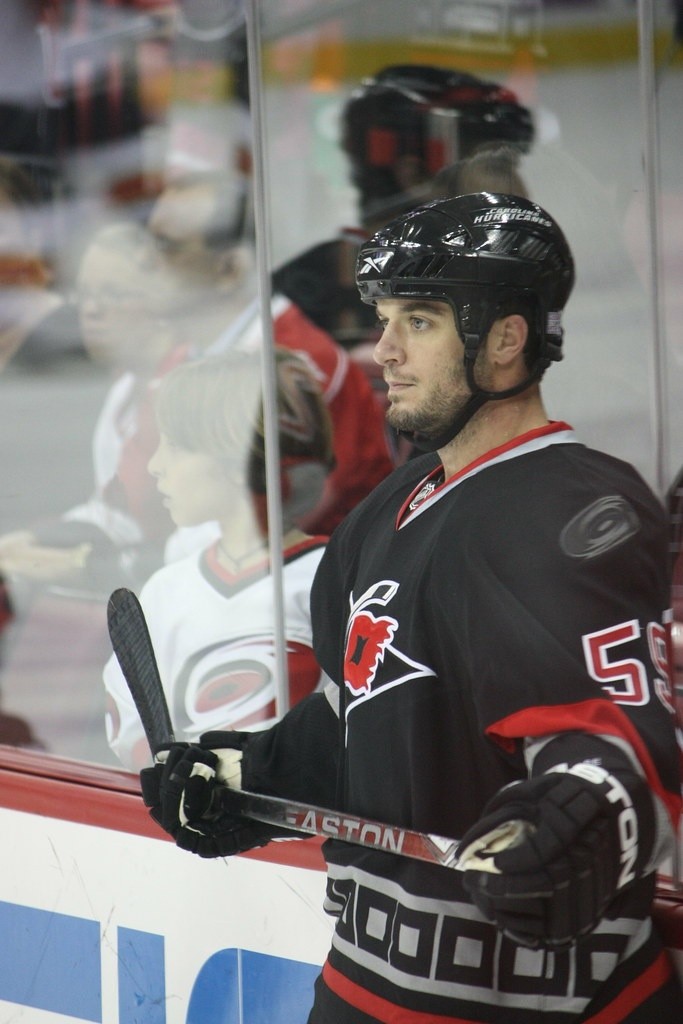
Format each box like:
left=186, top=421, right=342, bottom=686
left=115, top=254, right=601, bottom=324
left=354, top=192, right=575, bottom=361
left=338, top=60, right=538, bottom=212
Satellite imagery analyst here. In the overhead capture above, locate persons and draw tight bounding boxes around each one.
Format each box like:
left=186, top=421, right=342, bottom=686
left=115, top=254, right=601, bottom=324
left=243, top=58, right=536, bottom=557
left=139, top=187, right=683, bottom=1024
left=0, top=0, right=390, bottom=773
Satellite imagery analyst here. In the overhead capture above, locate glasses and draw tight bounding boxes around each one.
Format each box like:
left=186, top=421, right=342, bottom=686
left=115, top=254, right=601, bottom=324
left=67, top=283, right=147, bottom=305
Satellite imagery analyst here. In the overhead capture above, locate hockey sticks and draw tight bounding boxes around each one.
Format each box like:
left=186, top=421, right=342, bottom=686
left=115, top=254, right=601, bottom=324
left=105, top=587, right=658, bottom=918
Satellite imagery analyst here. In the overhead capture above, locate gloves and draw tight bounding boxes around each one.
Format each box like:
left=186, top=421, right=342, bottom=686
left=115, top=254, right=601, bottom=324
left=455, top=732, right=657, bottom=950
left=139, top=691, right=344, bottom=859
